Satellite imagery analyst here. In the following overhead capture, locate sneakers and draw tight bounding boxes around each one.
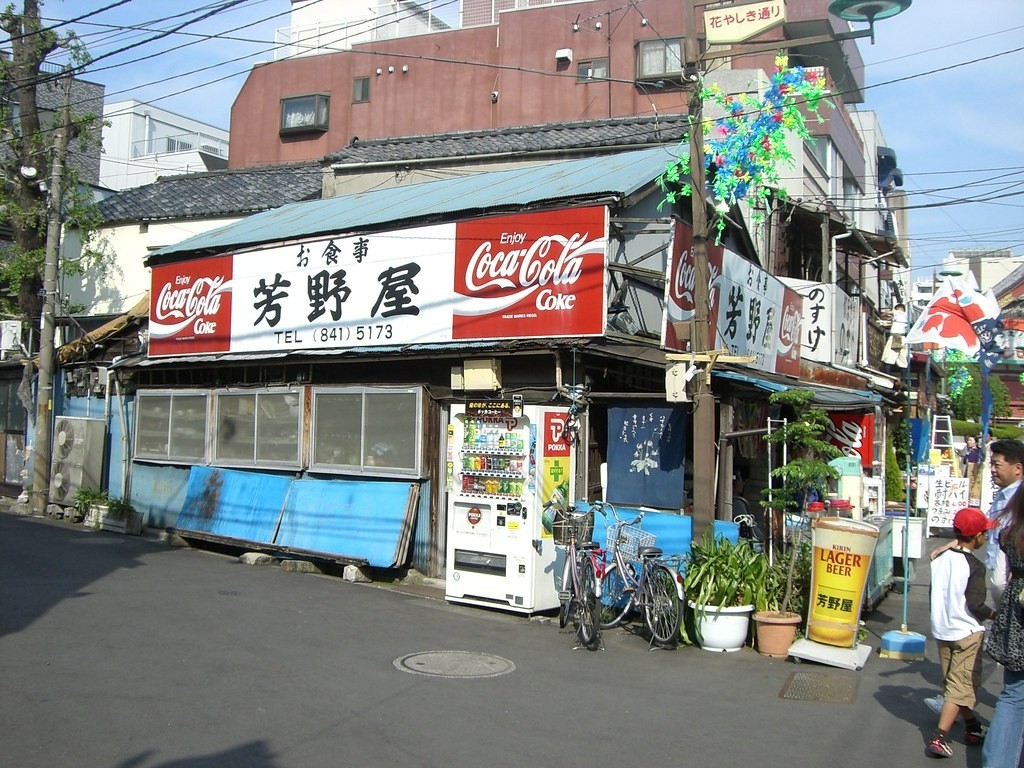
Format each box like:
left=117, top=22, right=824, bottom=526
left=964, top=720, right=989, bottom=745
left=929, top=736, right=953, bottom=757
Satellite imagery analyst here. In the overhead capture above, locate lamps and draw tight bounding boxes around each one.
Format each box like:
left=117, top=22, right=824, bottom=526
left=556, top=48, right=573, bottom=65
left=571, top=24, right=580, bottom=32
left=641, top=18, right=648, bottom=27
left=656, top=78, right=690, bottom=91
left=594, top=22, right=604, bottom=30
left=376, top=64, right=409, bottom=74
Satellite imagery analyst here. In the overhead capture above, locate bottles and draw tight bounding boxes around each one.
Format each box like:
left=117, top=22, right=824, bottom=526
left=460, top=402, right=526, bottom=497
left=807, top=499, right=852, bottom=523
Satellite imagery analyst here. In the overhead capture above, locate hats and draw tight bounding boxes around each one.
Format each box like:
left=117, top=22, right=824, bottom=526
left=955, top=508, right=1000, bottom=536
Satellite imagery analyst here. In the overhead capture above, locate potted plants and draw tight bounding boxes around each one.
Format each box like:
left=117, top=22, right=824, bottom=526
left=670, top=385, right=843, bottom=657
left=74, top=489, right=145, bottom=537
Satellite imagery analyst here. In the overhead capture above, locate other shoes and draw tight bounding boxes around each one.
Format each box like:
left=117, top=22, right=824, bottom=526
left=924, top=695, right=960, bottom=721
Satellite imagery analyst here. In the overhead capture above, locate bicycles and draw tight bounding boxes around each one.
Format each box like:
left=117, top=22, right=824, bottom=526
left=590, top=499, right=685, bottom=651
left=541, top=497, right=607, bottom=652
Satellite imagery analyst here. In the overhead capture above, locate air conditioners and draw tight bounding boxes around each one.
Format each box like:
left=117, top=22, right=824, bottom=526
left=47, top=415, right=106, bottom=510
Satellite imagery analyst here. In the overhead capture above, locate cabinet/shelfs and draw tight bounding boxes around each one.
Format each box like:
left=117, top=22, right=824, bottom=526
left=131, top=381, right=434, bottom=480
left=862, top=519, right=894, bottom=611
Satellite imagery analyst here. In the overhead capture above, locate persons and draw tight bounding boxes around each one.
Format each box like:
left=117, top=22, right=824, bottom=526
left=960, top=428, right=996, bottom=502
left=923, top=439, right=1024, bottom=714
left=982, top=481, right=1024, bottom=768
left=926, top=508, right=1001, bottom=757
left=881, top=303, right=912, bottom=377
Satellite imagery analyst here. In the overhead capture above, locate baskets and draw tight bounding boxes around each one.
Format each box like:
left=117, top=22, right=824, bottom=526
left=553, top=509, right=594, bottom=545
left=606, top=523, right=656, bottom=563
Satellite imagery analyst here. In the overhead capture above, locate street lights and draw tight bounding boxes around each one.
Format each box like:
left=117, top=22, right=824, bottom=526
left=683, top=0, right=911, bottom=576
left=922, top=269, right=963, bottom=423
